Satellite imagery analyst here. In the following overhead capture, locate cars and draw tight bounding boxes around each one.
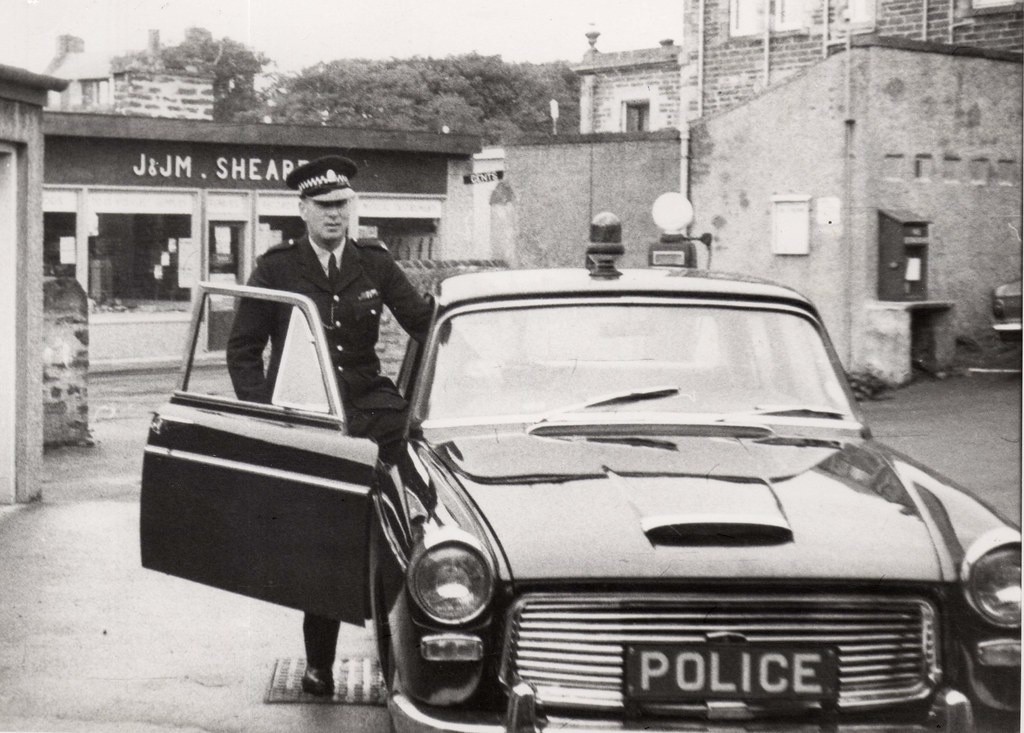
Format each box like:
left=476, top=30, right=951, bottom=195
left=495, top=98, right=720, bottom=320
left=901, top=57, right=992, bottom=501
left=139, top=212, right=1020, bottom=733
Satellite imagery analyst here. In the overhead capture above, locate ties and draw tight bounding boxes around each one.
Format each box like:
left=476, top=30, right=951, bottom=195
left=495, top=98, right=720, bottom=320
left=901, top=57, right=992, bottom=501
left=329, top=253, right=340, bottom=282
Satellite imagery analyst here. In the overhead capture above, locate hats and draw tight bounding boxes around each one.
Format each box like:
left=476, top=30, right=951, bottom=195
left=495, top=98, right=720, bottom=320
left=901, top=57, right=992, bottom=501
left=286, top=156, right=359, bottom=201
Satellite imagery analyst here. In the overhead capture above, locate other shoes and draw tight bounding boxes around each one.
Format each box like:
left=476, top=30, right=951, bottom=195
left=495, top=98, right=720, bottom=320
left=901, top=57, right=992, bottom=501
left=306, top=662, right=334, bottom=696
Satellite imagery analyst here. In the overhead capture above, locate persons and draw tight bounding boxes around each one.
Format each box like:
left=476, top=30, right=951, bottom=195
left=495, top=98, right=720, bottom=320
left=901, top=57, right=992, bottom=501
left=226, top=156, right=431, bottom=694
